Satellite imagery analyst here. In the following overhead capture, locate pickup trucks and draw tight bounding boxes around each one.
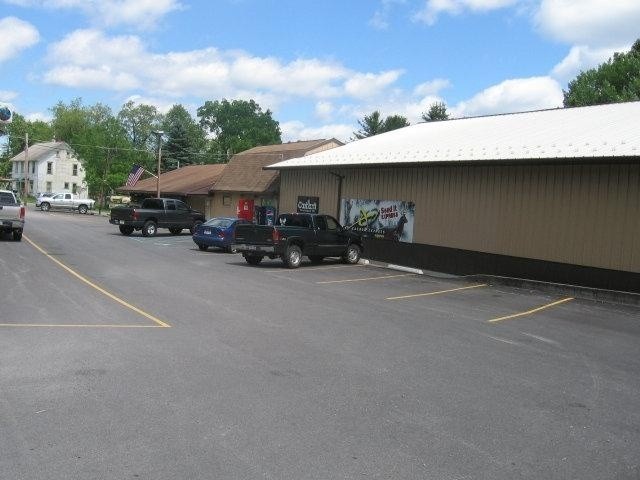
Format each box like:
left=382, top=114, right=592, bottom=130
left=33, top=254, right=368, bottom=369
left=36, top=193, right=96, bottom=214
left=232, top=212, right=365, bottom=269
left=108, top=197, right=206, bottom=238
left=0, top=189, right=25, bottom=242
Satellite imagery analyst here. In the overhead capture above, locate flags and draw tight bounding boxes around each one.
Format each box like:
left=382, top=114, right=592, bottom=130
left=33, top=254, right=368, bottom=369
left=126, top=164, right=145, bottom=188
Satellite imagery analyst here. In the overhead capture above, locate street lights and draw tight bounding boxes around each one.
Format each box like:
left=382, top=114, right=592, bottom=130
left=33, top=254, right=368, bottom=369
left=154, top=131, right=165, bottom=196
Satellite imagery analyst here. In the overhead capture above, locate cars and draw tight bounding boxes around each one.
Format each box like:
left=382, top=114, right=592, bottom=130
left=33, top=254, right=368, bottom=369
left=192, top=217, right=253, bottom=255
